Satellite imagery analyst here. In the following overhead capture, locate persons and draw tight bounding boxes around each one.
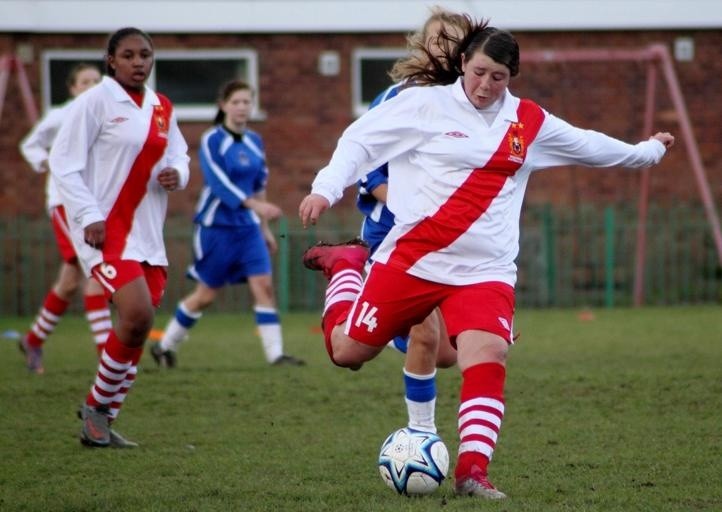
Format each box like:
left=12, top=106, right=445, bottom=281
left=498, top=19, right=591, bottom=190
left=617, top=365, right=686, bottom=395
left=16, top=63, right=114, bottom=375
left=47, top=26, right=192, bottom=447
left=148, top=77, right=309, bottom=368
left=295, top=25, right=679, bottom=502
left=354, top=8, right=474, bottom=433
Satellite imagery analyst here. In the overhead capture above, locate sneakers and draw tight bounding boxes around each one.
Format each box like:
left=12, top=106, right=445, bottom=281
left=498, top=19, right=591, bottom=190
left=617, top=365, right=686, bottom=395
left=302, top=239, right=371, bottom=274
left=76, top=400, right=111, bottom=448
left=269, top=355, right=307, bottom=369
left=19, top=332, right=44, bottom=375
left=454, top=464, right=508, bottom=500
left=79, top=425, right=139, bottom=449
left=151, top=342, right=178, bottom=373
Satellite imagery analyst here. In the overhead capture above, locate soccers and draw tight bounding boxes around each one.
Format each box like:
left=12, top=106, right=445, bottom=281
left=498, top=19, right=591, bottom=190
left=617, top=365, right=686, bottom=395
left=378, top=426, right=450, bottom=498
